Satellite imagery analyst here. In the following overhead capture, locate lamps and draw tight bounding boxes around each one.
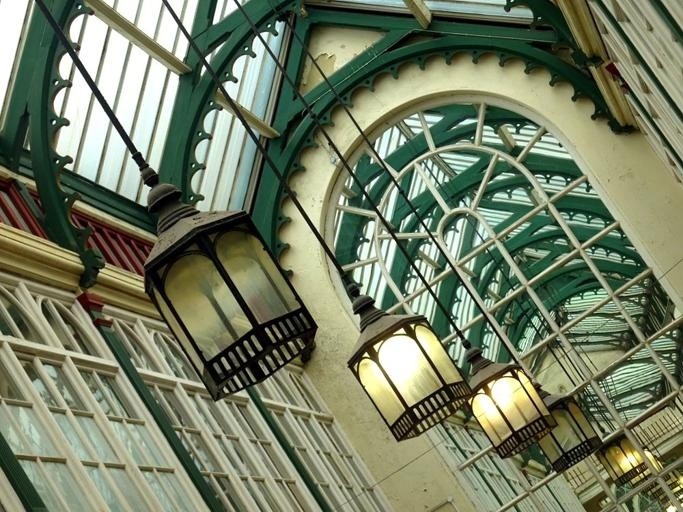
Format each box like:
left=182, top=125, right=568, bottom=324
left=161, top=1, right=472, bottom=442
left=235, top=1, right=559, bottom=459
left=274, top=3, right=604, bottom=472
left=422, top=156, right=648, bottom=488
left=37, top=0, right=318, bottom=402
